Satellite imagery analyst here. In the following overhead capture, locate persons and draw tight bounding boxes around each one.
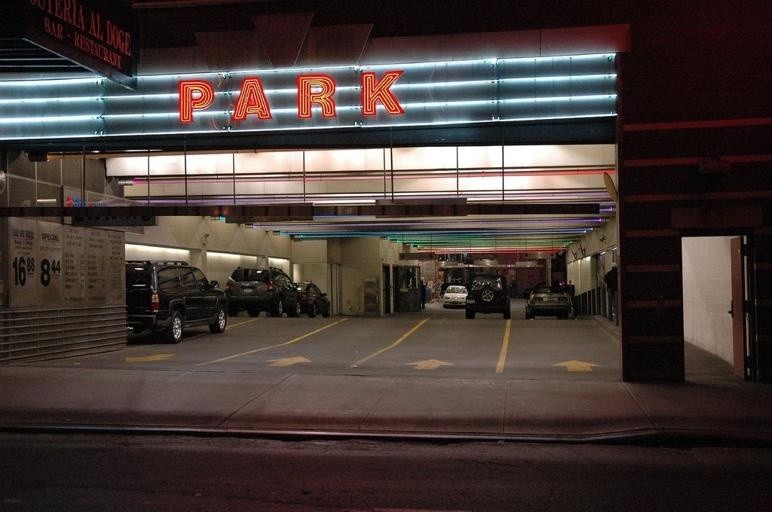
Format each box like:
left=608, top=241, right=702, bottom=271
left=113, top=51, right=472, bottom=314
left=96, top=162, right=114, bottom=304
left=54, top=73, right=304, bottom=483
left=559, top=280, right=575, bottom=306
left=510, top=277, right=517, bottom=298
left=604, top=263, right=618, bottom=317
left=418, top=277, right=428, bottom=308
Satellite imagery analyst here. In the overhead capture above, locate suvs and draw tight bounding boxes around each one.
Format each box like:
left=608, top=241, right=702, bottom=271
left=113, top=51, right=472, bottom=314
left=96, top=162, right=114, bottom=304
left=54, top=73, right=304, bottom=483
left=465, top=274, right=510, bottom=320
left=224, top=264, right=302, bottom=318
left=125, top=261, right=230, bottom=343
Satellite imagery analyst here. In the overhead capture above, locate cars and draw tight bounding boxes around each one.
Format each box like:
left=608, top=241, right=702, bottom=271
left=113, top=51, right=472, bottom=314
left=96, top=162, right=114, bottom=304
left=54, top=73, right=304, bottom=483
left=292, top=281, right=330, bottom=318
left=526, top=287, right=571, bottom=320
left=442, top=285, right=468, bottom=305
